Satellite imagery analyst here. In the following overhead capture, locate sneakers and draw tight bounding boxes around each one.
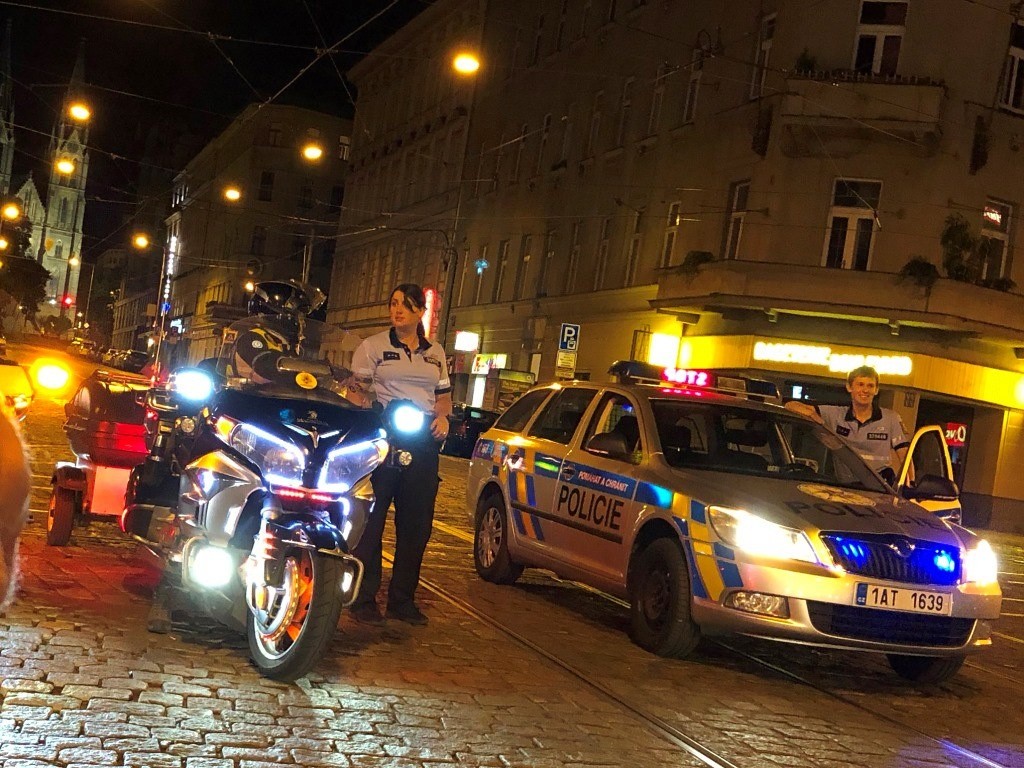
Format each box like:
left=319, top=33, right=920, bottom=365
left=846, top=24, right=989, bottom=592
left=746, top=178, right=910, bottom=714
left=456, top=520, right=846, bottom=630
left=348, top=598, right=386, bottom=625
left=386, top=599, right=430, bottom=624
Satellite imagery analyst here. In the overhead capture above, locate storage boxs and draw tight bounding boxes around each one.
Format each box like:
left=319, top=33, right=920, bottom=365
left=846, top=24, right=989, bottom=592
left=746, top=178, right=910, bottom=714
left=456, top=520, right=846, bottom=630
left=124, top=462, right=180, bottom=537
left=143, top=388, right=185, bottom=462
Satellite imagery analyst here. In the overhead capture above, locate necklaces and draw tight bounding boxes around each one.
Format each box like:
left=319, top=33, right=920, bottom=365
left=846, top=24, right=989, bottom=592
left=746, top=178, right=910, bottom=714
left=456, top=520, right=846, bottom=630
left=409, top=335, right=418, bottom=349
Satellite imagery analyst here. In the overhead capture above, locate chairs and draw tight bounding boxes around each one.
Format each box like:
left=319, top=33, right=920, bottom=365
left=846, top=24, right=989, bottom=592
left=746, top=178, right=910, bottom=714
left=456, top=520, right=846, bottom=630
left=559, top=410, right=767, bottom=470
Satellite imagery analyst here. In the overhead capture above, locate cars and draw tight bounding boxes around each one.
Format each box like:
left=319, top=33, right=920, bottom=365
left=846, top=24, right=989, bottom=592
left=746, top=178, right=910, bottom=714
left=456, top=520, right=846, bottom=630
left=86, top=346, right=127, bottom=368
left=466, top=360, right=1002, bottom=688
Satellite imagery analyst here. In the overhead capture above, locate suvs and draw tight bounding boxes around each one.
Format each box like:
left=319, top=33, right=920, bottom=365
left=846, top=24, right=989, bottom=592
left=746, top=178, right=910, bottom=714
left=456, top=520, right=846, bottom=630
left=122, top=349, right=148, bottom=373
left=438, top=401, right=501, bottom=458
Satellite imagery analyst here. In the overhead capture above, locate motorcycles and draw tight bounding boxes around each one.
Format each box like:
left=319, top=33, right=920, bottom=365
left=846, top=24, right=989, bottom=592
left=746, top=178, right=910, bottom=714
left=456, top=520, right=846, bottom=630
left=46, top=314, right=425, bottom=683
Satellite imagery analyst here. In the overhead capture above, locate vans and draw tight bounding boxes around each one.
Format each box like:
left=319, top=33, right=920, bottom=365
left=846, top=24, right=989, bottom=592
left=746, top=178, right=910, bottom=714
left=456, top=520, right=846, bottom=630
left=67, top=336, right=97, bottom=358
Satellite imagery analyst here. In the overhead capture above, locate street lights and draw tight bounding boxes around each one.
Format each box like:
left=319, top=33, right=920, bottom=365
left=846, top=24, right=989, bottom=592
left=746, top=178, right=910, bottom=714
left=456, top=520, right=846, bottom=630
left=69, top=258, right=96, bottom=338
left=34, top=94, right=94, bottom=316
left=135, top=235, right=166, bottom=328
left=226, top=188, right=317, bottom=358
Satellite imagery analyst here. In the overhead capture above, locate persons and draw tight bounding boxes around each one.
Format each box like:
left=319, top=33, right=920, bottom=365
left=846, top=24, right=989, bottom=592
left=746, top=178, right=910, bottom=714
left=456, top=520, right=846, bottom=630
left=785, top=367, right=915, bottom=487
left=147, top=279, right=327, bottom=634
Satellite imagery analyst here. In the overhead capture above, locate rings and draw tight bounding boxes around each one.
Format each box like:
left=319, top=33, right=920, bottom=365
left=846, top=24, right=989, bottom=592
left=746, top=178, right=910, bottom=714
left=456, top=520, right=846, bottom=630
left=346, top=284, right=452, bottom=627
left=443, top=432, right=447, bottom=435
